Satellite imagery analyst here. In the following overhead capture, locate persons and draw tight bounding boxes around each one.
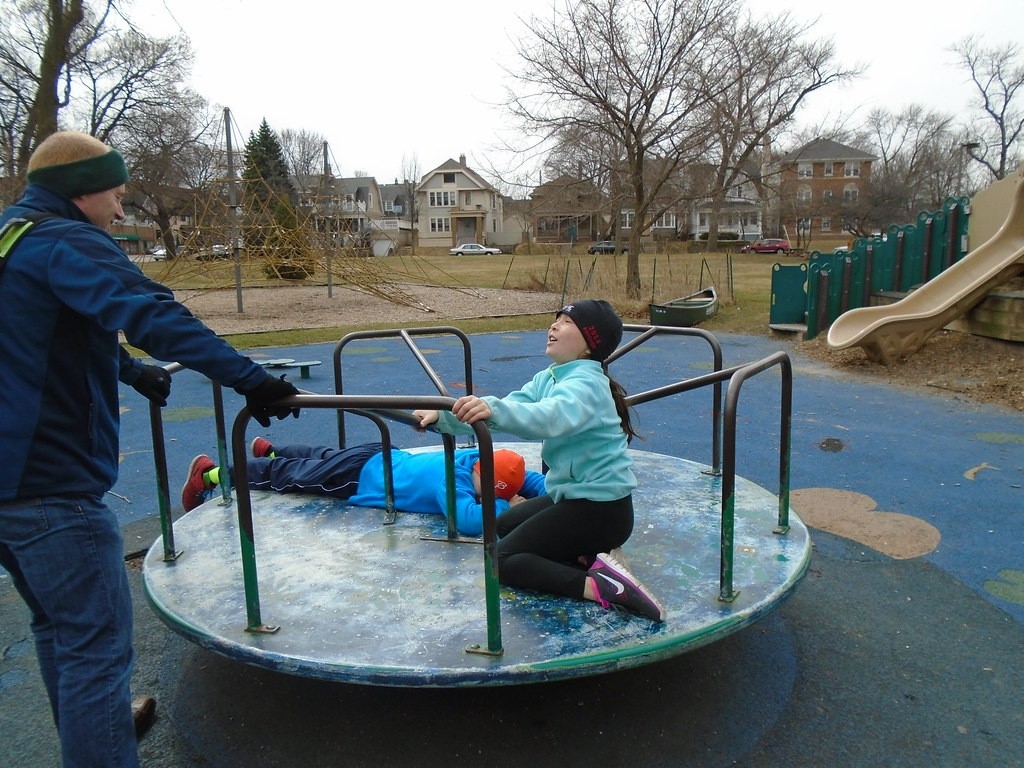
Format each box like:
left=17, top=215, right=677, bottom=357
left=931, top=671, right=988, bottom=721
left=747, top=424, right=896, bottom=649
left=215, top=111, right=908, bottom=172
left=182, top=437, right=546, bottom=536
left=0, top=132, right=300, bottom=768
left=411, top=298, right=665, bottom=623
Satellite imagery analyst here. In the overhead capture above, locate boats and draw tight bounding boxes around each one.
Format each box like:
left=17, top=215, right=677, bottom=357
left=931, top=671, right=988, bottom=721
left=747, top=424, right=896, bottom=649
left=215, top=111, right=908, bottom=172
left=648, top=286, right=718, bottom=328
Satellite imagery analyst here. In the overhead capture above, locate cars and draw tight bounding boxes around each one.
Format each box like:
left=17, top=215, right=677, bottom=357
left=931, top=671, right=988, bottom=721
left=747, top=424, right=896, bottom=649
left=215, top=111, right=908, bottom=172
left=448, top=243, right=502, bottom=257
left=587, top=240, right=629, bottom=256
left=151, top=243, right=206, bottom=262
left=196, top=249, right=234, bottom=262
left=740, top=238, right=788, bottom=255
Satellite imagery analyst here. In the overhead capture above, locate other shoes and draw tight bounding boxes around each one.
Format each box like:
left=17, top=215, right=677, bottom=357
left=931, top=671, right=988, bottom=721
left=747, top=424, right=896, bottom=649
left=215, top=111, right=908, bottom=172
left=131, top=695, right=156, bottom=735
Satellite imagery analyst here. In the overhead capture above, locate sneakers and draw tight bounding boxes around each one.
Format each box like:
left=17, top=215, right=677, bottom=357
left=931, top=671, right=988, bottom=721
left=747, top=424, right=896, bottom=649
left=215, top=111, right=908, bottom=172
left=182, top=454, right=215, bottom=513
left=250, top=437, right=272, bottom=458
left=586, top=552, right=666, bottom=622
left=610, top=548, right=631, bottom=573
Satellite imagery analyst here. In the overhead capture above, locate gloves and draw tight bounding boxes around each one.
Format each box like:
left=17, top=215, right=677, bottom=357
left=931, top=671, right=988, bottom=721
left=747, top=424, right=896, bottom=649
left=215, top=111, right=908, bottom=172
left=245, top=373, right=301, bottom=427
left=132, top=366, right=171, bottom=407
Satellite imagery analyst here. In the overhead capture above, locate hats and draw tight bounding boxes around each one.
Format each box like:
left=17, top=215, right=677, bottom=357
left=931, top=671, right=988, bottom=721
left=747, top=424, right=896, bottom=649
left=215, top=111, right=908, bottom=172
left=557, top=300, right=623, bottom=362
left=474, top=449, right=525, bottom=500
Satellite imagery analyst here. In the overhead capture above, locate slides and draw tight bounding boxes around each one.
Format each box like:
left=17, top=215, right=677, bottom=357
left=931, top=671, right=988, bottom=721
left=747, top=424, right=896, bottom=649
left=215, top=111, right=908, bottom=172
left=827, top=178, right=1024, bottom=367
